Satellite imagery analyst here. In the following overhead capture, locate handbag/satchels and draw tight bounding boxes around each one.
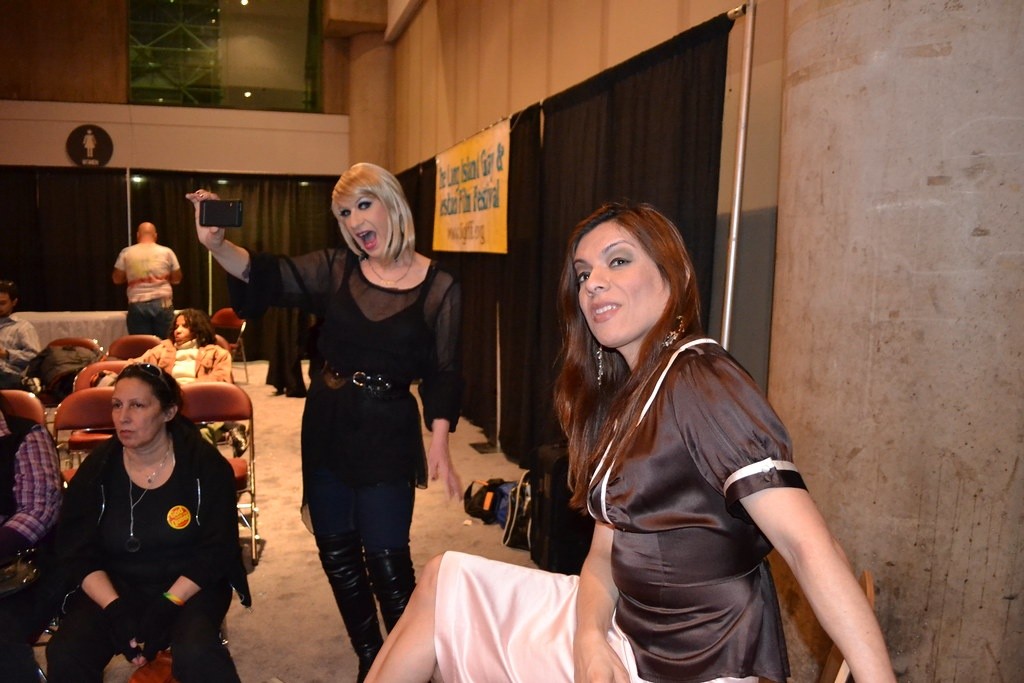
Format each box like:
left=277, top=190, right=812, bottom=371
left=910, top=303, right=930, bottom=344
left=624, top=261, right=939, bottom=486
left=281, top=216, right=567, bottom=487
left=0, top=560, right=39, bottom=599
left=465, top=480, right=503, bottom=524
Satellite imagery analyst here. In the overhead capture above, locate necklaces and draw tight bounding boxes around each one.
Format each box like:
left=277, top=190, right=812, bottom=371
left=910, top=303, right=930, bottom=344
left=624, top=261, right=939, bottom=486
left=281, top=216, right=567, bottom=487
left=369, top=254, right=414, bottom=285
left=124, top=439, right=172, bottom=554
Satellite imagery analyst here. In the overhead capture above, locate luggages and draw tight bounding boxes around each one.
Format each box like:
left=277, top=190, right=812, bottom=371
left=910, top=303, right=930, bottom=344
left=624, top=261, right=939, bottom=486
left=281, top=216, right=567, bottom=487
left=531, top=439, right=598, bottom=573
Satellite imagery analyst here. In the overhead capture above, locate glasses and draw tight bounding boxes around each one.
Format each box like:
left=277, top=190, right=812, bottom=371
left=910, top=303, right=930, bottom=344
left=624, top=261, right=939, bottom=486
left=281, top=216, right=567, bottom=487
left=117, top=363, right=172, bottom=395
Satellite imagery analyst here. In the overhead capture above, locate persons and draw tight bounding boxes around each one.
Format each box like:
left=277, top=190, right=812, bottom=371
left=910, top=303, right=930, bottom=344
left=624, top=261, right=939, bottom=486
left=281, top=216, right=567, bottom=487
left=0, top=390, right=60, bottom=683
left=112, top=222, right=181, bottom=342
left=365, top=201, right=898, bottom=683
left=128, top=308, right=249, bottom=457
left=46, top=363, right=252, bottom=683
left=183, top=162, right=460, bottom=683
left=0, top=280, right=42, bottom=393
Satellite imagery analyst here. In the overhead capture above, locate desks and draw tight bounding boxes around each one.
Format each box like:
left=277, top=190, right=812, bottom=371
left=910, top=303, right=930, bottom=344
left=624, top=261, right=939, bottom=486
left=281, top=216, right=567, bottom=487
left=9, top=310, right=130, bottom=354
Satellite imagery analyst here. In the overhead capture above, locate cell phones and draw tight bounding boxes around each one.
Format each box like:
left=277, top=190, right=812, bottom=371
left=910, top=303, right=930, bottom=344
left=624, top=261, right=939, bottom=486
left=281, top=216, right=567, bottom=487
left=200, top=199, right=242, bottom=227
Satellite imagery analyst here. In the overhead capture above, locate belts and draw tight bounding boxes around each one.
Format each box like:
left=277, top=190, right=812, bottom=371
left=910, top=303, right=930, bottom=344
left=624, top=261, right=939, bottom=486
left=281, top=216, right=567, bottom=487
left=324, top=361, right=407, bottom=392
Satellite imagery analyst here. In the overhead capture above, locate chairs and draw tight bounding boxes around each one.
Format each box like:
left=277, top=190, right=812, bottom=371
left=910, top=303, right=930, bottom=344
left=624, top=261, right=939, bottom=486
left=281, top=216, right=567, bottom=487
left=0, top=307, right=263, bottom=683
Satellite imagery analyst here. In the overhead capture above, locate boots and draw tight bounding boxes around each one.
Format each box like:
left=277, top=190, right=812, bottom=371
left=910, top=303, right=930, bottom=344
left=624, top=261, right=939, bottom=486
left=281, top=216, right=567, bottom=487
left=314, top=532, right=386, bottom=683
left=364, top=546, right=416, bottom=636
